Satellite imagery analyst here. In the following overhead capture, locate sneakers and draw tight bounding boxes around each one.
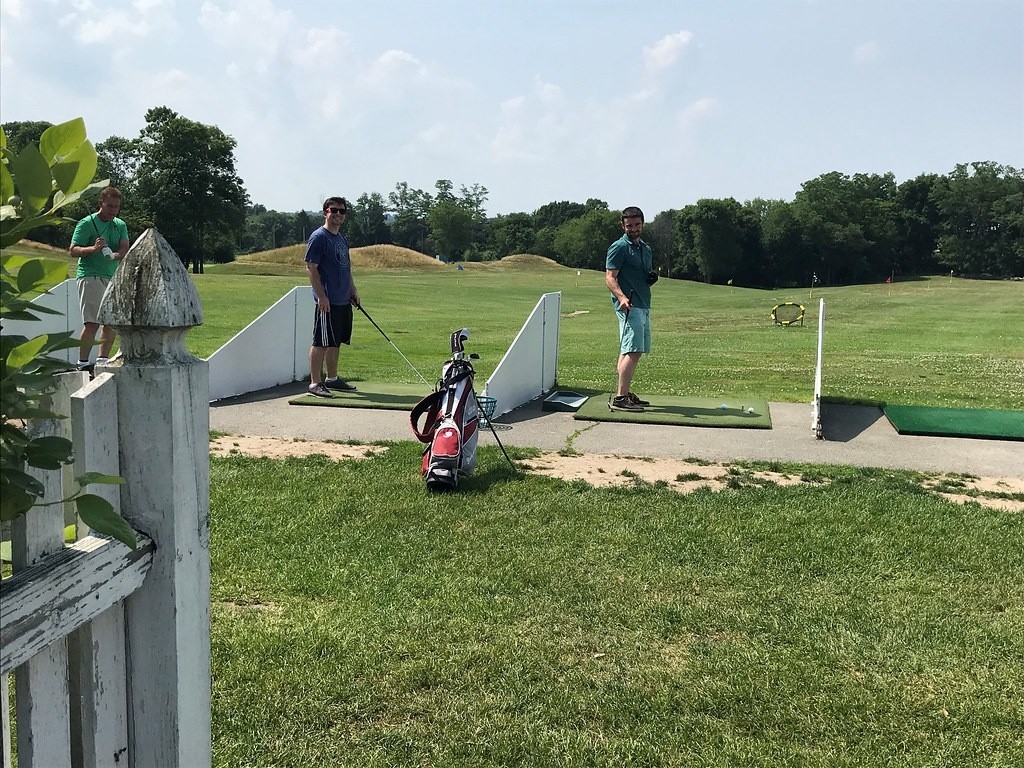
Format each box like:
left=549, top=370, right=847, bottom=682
left=628, top=393, right=649, bottom=407
left=324, top=376, right=356, bottom=392
left=307, top=382, right=336, bottom=399
left=611, top=396, right=644, bottom=412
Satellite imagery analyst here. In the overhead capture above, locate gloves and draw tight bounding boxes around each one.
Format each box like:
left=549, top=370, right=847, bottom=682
left=102, top=247, right=115, bottom=260
left=647, top=271, right=658, bottom=285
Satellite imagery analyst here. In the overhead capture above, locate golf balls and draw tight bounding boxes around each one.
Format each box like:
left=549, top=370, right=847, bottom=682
left=722, top=403, right=728, bottom=410
left=748, top=407, right=754, bottom=413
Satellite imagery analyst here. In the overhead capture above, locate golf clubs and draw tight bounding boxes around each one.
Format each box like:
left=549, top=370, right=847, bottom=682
left=350, top=297, right=436, bottom=393
left=606, top=288, right=635, bottom=413
left=476, top=399, right=516, bottom=471
left=467, top=352, right=480, bottom=363
left=84, top=202, right=110, bottom=259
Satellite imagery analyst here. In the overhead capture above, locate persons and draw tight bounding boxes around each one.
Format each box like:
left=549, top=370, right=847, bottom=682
left=69, top=187, right=130, bottom=372
left=304, top=197, right=361, bottom=399
left=605, top=207, right=658, bottom=413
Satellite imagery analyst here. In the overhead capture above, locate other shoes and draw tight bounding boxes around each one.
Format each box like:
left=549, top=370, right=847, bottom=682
left=76, top=365, right=93, bottom=379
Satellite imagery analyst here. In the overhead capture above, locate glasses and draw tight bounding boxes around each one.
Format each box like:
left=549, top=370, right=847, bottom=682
left=326, top=208, right=346, bottom=215
